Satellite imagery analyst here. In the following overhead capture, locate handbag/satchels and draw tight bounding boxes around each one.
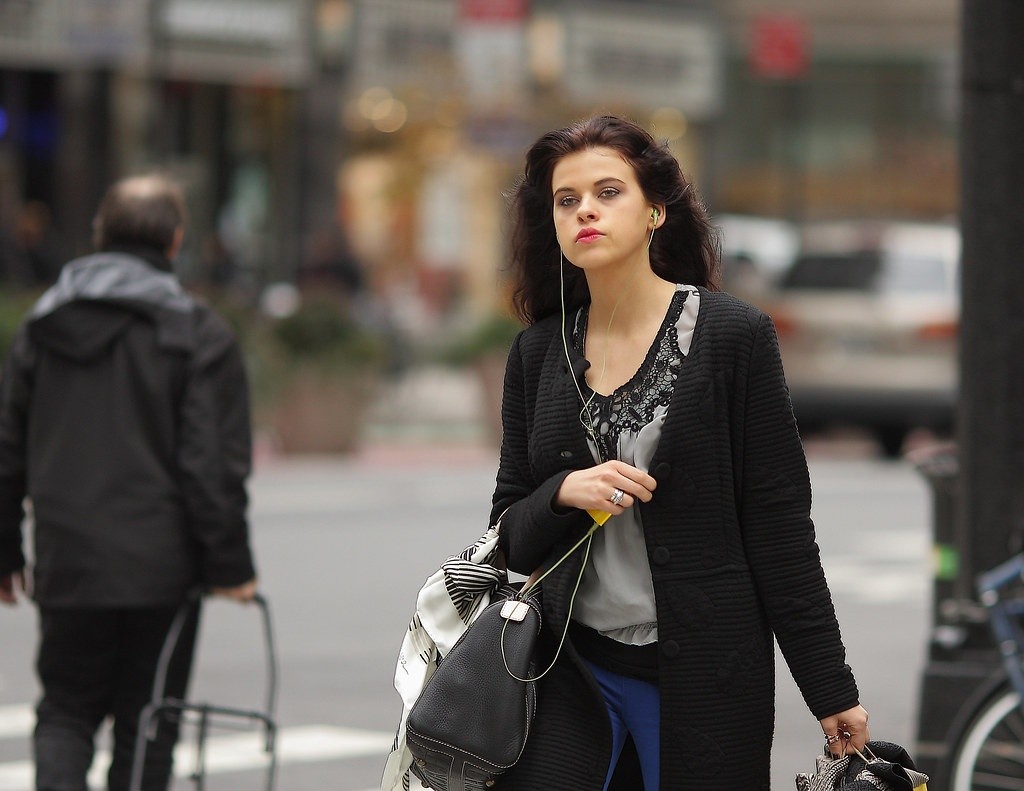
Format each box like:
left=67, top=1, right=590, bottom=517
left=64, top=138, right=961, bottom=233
left=795, top=741, right=930, bottom=791
left=406, top=561, right=543, bottom=791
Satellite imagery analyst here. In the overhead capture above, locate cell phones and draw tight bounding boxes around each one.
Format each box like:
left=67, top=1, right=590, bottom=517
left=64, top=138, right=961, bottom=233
left=585, top=509, right=612, bottom=525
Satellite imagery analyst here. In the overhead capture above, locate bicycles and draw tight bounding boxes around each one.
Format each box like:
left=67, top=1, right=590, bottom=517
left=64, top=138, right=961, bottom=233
left=901, top=439, right=1024, bottom=790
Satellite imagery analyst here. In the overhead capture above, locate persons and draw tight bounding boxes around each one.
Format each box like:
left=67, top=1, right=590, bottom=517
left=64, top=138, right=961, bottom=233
left=0, top=177, right=258, bottom=791
left=487, top=115, right=868, bottom=791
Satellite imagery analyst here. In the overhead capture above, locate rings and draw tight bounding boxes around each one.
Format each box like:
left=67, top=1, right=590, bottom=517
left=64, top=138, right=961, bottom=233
left=826, top=735, right=839, bottom=744
left=611, top=490, right=623, bottom=506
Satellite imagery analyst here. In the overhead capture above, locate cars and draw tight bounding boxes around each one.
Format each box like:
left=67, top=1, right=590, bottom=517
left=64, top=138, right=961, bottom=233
left=708, top=213, right=962, bottom=459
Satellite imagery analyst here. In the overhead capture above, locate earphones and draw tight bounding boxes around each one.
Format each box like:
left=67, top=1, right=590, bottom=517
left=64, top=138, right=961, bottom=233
left=651, top=207, right=660, bottom=227
